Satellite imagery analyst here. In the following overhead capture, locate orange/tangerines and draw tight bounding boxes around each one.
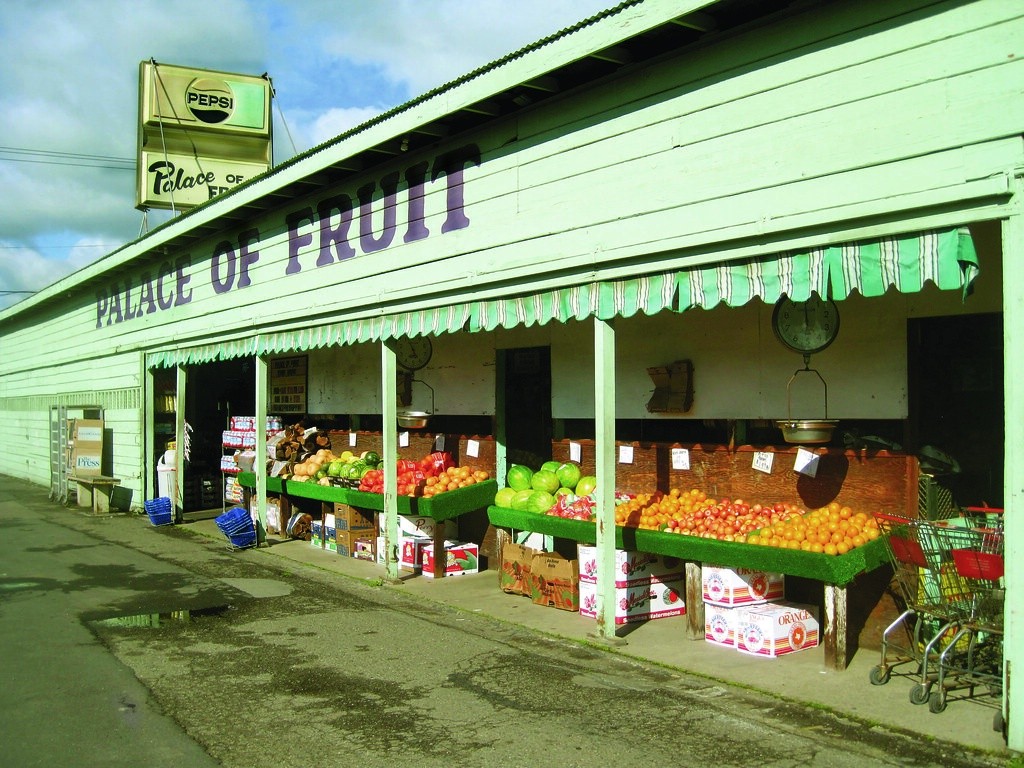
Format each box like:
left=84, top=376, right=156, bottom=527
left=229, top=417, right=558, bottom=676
left=613, top=489, right=717, bottom=531
left=358, top=452, right=488, bottom=497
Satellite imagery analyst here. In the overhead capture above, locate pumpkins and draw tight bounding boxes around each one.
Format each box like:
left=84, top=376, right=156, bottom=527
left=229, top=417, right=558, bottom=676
left=291, top=449, right=330, bottom=482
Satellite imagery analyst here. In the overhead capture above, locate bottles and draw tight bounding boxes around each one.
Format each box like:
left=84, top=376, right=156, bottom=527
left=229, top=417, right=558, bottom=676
left=223, top=416, right=282, bottom=449
left=220, top=455, right=243, bottom=472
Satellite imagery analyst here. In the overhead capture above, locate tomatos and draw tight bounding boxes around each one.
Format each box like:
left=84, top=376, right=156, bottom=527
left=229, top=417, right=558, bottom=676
left=746, top=501, right=889, bottom=556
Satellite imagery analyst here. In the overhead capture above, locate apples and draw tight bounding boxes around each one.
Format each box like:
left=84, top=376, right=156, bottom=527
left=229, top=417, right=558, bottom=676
left=662, top=497, right=807, bottom=543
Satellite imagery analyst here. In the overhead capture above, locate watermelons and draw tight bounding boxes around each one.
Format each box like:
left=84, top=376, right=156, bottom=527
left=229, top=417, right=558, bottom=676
left=303, top=451, right=400, bottom=484
left=494, top=461, right=596, bottom=514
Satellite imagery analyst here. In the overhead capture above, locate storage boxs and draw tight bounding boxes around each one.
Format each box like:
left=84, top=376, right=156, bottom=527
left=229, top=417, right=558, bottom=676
left=64, top=418, right=104, bottom=475
left=736, top=602, right=820, bottom=658
left=500, top=540, right=687, bottom=623
left=700, top=561, right=785, bottom=608
left=704, top=599, right=785, bottom=650
left=144, top=417, right=479, bottom=581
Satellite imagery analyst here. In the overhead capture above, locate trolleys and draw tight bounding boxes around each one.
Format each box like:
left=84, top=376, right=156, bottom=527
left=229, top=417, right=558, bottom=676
left=868, top=504, right=1012, bottom=733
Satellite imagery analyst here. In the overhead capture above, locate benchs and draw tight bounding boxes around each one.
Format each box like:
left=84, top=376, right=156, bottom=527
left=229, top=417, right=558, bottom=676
left=66, top=475, right=122, bottom=515
